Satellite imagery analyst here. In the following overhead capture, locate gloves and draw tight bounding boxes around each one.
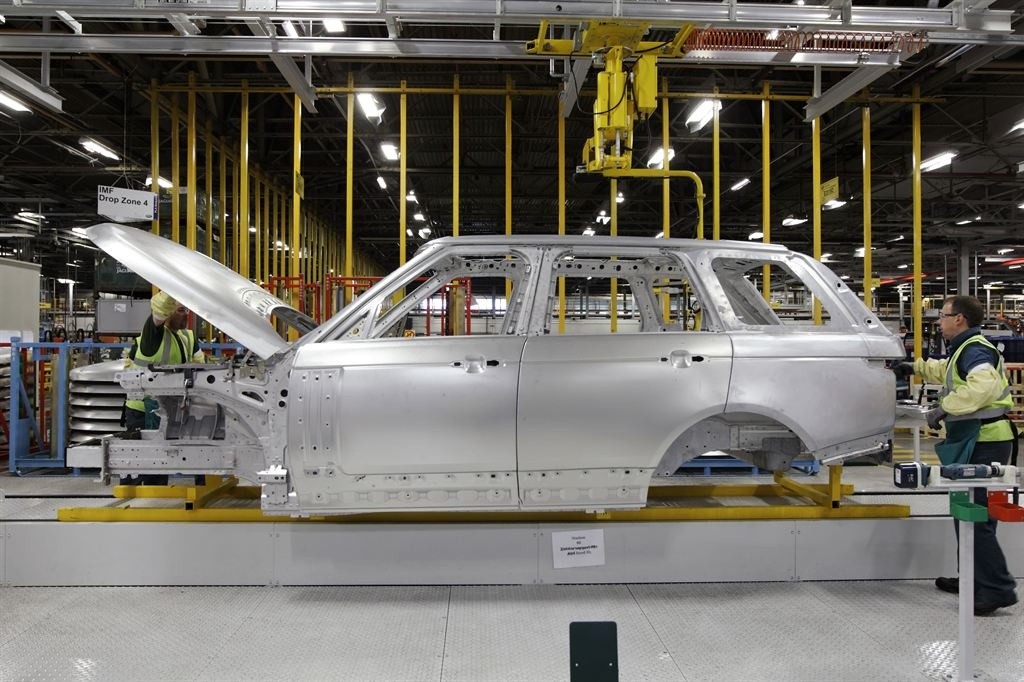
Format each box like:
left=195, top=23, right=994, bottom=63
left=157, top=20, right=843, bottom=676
left=893, top=362, right=915, bottom=376
left=926, top=407, right=948, bottom=430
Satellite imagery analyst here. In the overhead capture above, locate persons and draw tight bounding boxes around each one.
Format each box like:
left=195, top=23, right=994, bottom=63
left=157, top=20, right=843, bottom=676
left=894, top=296, right=1017, bottom=614
left=125, top=292, right=205, bottom=486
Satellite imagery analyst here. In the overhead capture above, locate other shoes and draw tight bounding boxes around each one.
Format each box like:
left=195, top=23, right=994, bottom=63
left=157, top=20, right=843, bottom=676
left=935, top=577, right=960, bottom=593
left=973, top=590, right=1018, bottom=616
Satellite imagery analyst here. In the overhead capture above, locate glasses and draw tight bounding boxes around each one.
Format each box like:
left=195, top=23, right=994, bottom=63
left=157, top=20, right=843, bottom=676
left=938, top=311, right=968, bottom=322
